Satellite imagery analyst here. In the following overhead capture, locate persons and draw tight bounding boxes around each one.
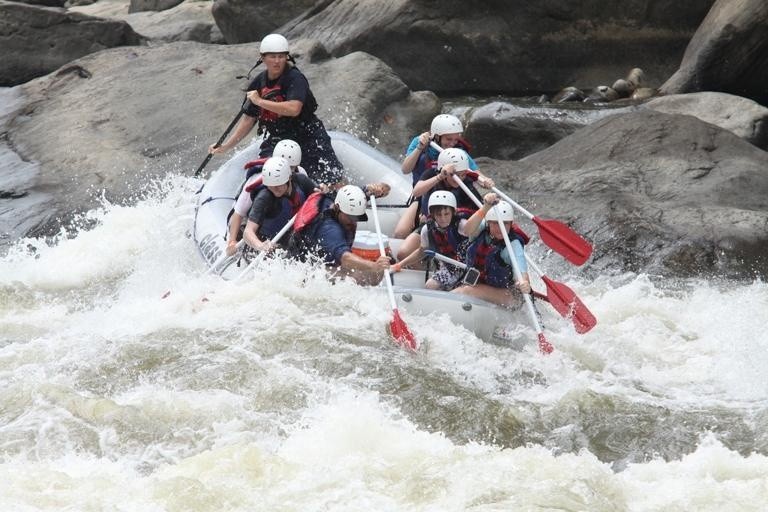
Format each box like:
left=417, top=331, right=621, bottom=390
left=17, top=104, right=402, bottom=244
left=392, top=112, right=495, bottom=239
left=395, top=147, right=486, bottom=270
left=424, top=192, right=535, bottom=312
left=387, top=190, right=486, bottom=292
left=285, top=180, right=394, bottom=274
left=224, top=139, right=330, bottom=257
left=207, top=32, right=353, bottom=193
left=241, top=156, right=332, bottom=258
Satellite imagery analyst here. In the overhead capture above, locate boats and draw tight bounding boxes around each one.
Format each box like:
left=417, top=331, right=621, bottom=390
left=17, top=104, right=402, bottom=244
left=196, top=129, right=532, bottom=351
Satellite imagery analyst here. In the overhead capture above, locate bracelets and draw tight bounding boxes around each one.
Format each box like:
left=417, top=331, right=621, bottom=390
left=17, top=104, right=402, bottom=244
left=416, top=145, right=424, bottom=150
left=227, top=240, right=237, bottom=247
left=436, top=175, right=443, bottom=182
left=394, top=263, right=402, bottom=273
left=473, top=209, right=489, bottom=220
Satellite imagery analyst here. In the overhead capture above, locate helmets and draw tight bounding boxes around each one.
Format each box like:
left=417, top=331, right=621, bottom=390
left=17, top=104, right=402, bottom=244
left=334, top=184, right=368, bottom=221
left=430, top=114, right=464, bottom=140
left=427, top=190, right=457, bottom=216
left=438, top=147, right=469, bottom=174
left=486, top=201, right=514, bottom=223
left=272, top=139, right=302, bottom=167
left=261, top=157, right=292, bottom=187
left=259, top=32, right=290, bottom=55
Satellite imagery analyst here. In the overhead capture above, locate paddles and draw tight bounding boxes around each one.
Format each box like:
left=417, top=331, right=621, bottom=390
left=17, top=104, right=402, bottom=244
left=452, top=174, right=597, bottom=334
left=493, top=205, right=554, bottom=356
left=429, top=140, right=592, bottom=265
left=370, top=194, right=416, bottom=351
